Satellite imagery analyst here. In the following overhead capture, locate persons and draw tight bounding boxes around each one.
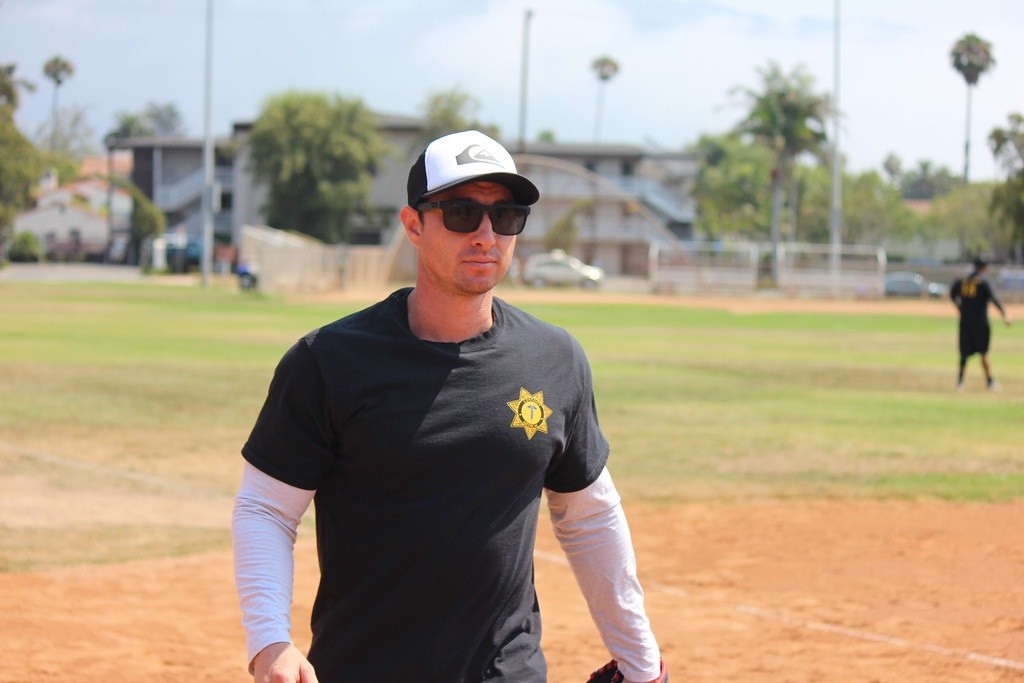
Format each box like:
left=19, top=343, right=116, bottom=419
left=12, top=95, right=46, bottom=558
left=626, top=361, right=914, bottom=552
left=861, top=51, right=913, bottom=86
left=232, top=130, right=668, bottom=682
left=948, top=256, right=1012, bottom=391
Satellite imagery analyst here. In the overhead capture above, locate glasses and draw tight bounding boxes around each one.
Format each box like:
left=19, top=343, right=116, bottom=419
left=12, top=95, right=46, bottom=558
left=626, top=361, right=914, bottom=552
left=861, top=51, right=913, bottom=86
left=416, top=198, right=530, bottom=235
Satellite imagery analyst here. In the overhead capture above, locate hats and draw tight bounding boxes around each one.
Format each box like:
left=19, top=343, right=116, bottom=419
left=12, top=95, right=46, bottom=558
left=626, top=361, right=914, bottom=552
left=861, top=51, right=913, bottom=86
left=408, top=130, right=540, bottom=206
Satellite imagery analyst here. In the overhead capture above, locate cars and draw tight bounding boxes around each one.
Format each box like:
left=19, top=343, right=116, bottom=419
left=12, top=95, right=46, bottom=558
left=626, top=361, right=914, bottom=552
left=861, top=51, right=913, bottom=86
left=522, top=248, right=607, bottom=292
left=884, top=271, right=947, bottom=300
